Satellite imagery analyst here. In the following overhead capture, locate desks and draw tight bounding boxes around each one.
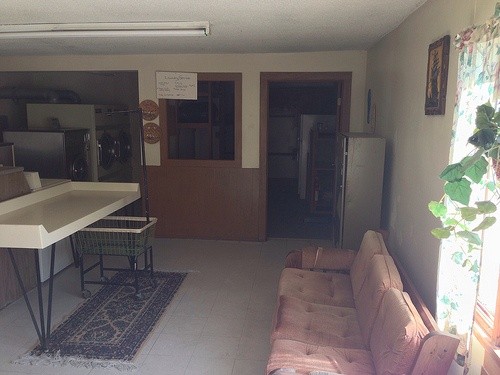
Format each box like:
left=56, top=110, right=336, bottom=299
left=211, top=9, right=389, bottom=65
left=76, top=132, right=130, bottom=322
left=0, top=181, right=142, bottom=353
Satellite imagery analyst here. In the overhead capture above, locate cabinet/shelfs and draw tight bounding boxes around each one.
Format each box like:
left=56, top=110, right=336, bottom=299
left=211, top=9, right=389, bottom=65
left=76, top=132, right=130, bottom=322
left=306, top=130, right=336, bottom=215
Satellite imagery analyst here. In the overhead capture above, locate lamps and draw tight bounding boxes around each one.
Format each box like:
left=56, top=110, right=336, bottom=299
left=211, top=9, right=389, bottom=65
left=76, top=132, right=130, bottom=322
left=0, top=20, right=210, bottom=39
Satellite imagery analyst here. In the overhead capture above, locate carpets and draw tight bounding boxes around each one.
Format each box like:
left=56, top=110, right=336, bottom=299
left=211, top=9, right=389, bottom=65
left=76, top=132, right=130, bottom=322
left=11, top=267, right=192, bottom=373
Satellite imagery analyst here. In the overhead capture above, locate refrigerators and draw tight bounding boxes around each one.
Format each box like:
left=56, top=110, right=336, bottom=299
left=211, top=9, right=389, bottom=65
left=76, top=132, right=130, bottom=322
left=298, top=114, right=338, bottom=200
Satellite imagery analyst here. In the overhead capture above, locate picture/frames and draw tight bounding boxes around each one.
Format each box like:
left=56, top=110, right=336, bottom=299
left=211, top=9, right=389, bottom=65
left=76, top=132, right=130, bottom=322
left=424, top=35, right=451, bottom=115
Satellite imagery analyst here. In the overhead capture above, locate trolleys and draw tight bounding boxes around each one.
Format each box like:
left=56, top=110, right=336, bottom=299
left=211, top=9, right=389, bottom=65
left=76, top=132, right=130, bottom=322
left=68, top=215, right=156, bottom=301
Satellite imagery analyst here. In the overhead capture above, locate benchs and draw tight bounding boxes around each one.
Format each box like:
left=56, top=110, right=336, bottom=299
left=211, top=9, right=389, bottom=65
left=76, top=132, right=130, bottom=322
left=265, top=228, right=460, bottom=375
